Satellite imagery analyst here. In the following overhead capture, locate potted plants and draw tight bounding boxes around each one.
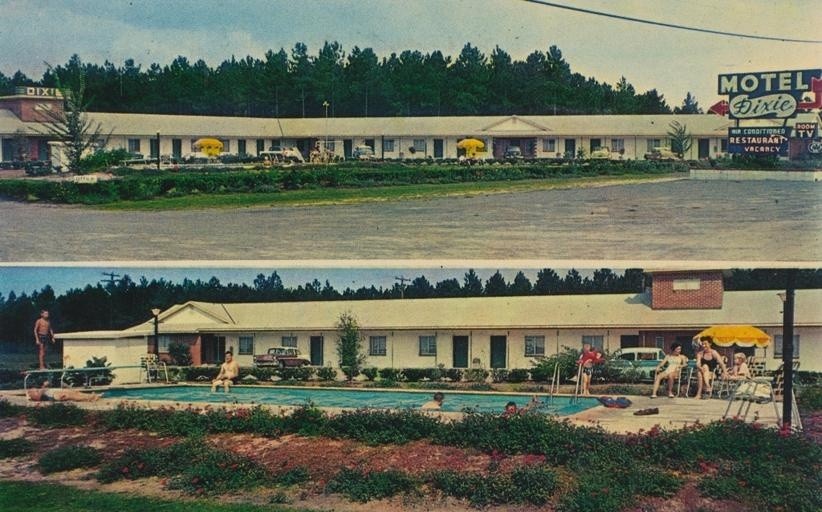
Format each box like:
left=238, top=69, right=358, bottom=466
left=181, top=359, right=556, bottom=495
left=62, top=356, right=117, bottom=387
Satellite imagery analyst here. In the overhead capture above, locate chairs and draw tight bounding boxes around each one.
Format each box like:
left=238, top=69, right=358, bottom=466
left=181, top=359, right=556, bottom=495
left=651, top=354, right=800, bottom=430
left=139, top=353, right=169, bottom=384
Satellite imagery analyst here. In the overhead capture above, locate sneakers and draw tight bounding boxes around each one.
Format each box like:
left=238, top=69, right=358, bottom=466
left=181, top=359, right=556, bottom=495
left=633, top=408, right=659, bottom=415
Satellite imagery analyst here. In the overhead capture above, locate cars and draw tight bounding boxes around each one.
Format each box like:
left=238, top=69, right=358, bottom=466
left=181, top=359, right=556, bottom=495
left=599, top=345, right=696, bottom=382
left=253, top=347, right=311, bottom=369
left=352, top=145, right=373, bottom=159
left=259, top=145, right=289, bottom=159
left=504, top=146, right=521, bottom=158
left=644, top=146, right=674, bottom=161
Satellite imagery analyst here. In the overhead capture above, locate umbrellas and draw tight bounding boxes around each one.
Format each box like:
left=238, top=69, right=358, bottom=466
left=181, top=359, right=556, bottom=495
left=455, top=138, right=484, bottom=148
left=194, top=138, right=224, bottom=149
left=692, top=325, right=771, bottom=353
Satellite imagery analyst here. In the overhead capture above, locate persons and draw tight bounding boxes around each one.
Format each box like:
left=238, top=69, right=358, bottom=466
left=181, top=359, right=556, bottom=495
left=29, top=379, right=103, bottom=401
left=34, top=307, right=56, bottom=369
left=649, top=343, right=687, bottom=397
left=692, top=335, right=728, bottom=400
left=575, top=343, right=601, bottom=395
left=422, top=392, right=443, bottom=408
left=211, top=350, right=241, bottom=393
left=503, top=399, right=532, bottom=414
left=722, top=352, right=751, bottom=400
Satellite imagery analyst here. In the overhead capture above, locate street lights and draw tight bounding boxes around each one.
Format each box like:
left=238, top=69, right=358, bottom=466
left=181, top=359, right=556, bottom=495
left=151, top=308, right=161, bottom=379
left=322, top=100, right=330, bottom=150
